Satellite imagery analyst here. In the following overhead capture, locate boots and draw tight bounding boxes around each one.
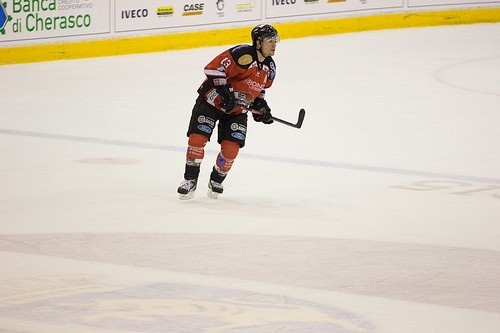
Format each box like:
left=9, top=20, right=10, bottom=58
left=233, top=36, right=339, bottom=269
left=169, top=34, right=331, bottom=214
left=177, top=164, right=199, bottom=200
left=208, top=165, right=227, bottom=199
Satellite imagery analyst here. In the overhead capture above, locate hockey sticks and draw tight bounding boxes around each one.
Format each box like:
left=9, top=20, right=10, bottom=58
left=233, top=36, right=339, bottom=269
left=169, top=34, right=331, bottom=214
left=219, top=101, right=306, bottom=129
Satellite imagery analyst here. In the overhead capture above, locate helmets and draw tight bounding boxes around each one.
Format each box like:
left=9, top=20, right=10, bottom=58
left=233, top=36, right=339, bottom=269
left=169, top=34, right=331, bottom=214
left=251, top=24, right=280, bottom=46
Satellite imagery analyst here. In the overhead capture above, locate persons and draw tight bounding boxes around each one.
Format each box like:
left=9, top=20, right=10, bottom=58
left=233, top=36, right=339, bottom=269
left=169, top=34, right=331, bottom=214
left=177, top=24, right=281, bottom=201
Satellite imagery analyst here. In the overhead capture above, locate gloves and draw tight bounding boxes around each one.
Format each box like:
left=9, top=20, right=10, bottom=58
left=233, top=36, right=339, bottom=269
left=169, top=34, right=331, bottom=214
left=249, top=97, right=273, bottom=124
left=216, top=84, right=236, bottom=112
